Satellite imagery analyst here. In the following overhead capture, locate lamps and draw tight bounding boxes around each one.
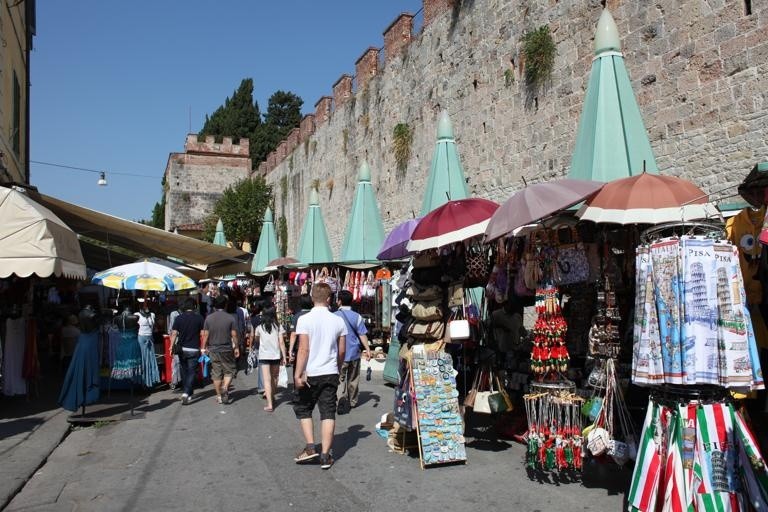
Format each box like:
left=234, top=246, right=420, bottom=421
left=97, top=170, right=109, bottom=187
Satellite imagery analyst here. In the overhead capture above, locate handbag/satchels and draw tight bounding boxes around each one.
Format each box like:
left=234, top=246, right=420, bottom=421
left=358, top=342, right=364, bottom=353
left=581, top=423, right=638, bottom=467
left=473, top=346, right=496, bottom=367
left=445, top=281, right=478, bottom=344
left=395, top=249, right=446, bottom=360
left=581, top=361, right=607, bottom=421
left=463, top=387, right=513, bottom=413
left=277, top=365, right=288, bottom=388
left=393, top=388, right=417, bottom=431
left=263, top=284, right=273, bottom=292
left=464, top=222, right=600, bottom=302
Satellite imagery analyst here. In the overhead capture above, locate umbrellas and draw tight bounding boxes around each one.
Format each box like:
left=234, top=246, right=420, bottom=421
left=297, top=185, right=336, bottom=264
left=262, top=258, right=300, bottom=268
left=338, top=160, right=400, bottom=266
left=405, top=193, right=500, bottom=250
left=377, top=211, right=435, bottom=262
left=92, top=258, right=195, bottom=310
left=212, top=219, right=235, bottom=280
left=419, top=109, right=472, bottom=218
left=739, top=162, right=768, bottom=208
left=250, top=205, right=282, bottom=276
left=567, top=6, right=660, bottom=182
left=482, top=176, right=608, bottom=244
left=574, top=159, right=721, bottom=225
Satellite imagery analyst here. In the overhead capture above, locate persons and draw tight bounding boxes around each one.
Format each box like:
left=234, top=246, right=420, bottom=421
left=58, top=305, right=101, bottom=411
left=163, top=284, right=373, bottom=416
left=291, top=282, right=349, bottom=469
left=110, top=308, right=141, bottom=380
left=135, top=305, right=160, bottom=388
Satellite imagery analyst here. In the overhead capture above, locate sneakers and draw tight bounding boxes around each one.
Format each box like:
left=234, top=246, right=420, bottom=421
left=320, top=453, right=333, bottom=469
left=294, top=448, right=318, bottom=463
left=181, top=393, right=190, bottom=404
left=336, top=397, right=357, bottom=415
left=215, top=390, right=230, bottom=403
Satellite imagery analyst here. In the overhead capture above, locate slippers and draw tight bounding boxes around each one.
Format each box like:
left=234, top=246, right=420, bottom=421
left=258, top=394, right=277, bottom=412
